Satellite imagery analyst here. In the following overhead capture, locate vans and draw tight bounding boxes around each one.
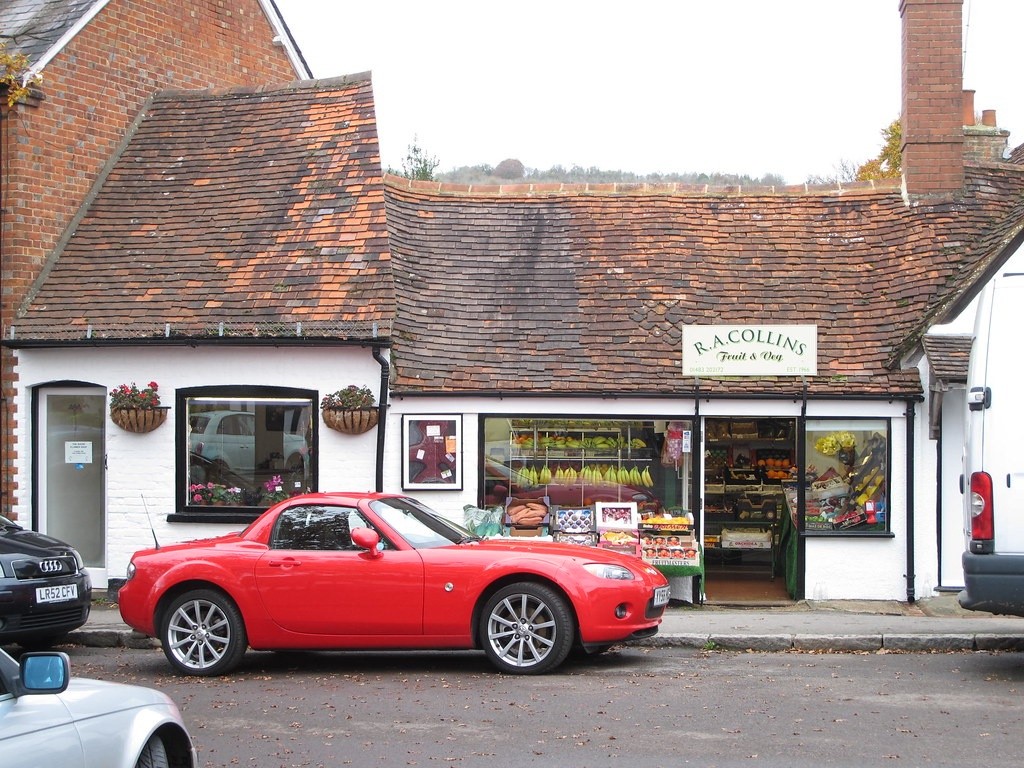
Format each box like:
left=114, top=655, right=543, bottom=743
left=958, top=273, right=1024, bottom=618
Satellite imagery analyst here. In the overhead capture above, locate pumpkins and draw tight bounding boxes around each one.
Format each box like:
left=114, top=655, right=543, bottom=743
left=504, top=501, right=548, bottom=532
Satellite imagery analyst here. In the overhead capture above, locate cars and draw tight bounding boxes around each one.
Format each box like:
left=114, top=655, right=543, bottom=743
left=0, top=514, right=198, bottom=768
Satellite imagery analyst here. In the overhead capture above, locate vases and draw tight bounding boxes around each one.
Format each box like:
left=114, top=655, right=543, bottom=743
left=110, top=406, right=171, bottom=434
left=321, top=407, right=382, bottom=434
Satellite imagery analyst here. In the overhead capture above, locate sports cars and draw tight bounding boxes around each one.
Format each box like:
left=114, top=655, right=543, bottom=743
left=119, top=492, right=671, bottom=676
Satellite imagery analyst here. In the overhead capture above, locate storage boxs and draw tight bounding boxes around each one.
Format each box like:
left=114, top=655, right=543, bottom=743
left=502, top=495, right=700, bottom=567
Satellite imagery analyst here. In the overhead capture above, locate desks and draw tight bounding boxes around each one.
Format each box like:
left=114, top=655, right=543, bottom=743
left=650, top=541, right=706, bottom=607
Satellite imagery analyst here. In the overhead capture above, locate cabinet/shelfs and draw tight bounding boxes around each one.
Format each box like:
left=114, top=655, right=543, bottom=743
left=509, top=427, right=622, bottom=506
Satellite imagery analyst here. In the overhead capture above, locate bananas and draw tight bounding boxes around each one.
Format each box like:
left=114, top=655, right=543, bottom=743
left=503, top=432, right=654, bottom=496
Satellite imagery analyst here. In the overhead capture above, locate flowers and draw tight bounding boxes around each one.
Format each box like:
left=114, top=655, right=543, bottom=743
left=190, top=475, right=311, bottom=506
left=109, top=381, right=161, bottom=411
left=320, top=385, right=376, bottom=413
